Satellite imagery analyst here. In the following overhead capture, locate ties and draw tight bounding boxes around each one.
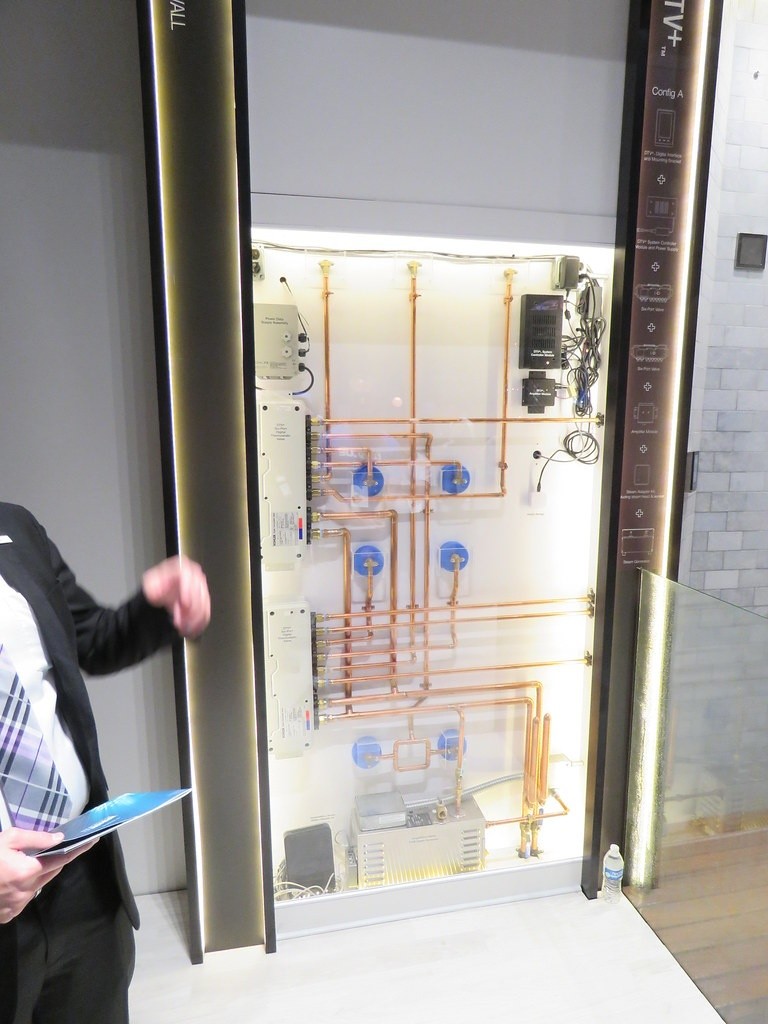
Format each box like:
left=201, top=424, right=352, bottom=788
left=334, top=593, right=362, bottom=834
left=0, top=646, right=72, bottom=835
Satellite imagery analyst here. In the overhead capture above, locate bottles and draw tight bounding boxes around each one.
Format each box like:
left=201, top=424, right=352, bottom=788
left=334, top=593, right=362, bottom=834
left=601, top=844, right=624, bottom=904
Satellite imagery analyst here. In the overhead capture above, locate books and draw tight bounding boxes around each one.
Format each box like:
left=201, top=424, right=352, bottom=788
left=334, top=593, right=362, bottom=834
left=27, top=788, right=194, bottom=858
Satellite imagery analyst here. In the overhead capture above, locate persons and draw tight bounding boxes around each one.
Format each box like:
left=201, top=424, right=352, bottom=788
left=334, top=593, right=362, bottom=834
left=0, top=500, right=211, bottom=1024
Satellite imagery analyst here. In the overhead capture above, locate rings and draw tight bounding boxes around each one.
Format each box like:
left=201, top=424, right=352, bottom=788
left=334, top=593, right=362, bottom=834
left=35, top=889, right=41, bottom=898
